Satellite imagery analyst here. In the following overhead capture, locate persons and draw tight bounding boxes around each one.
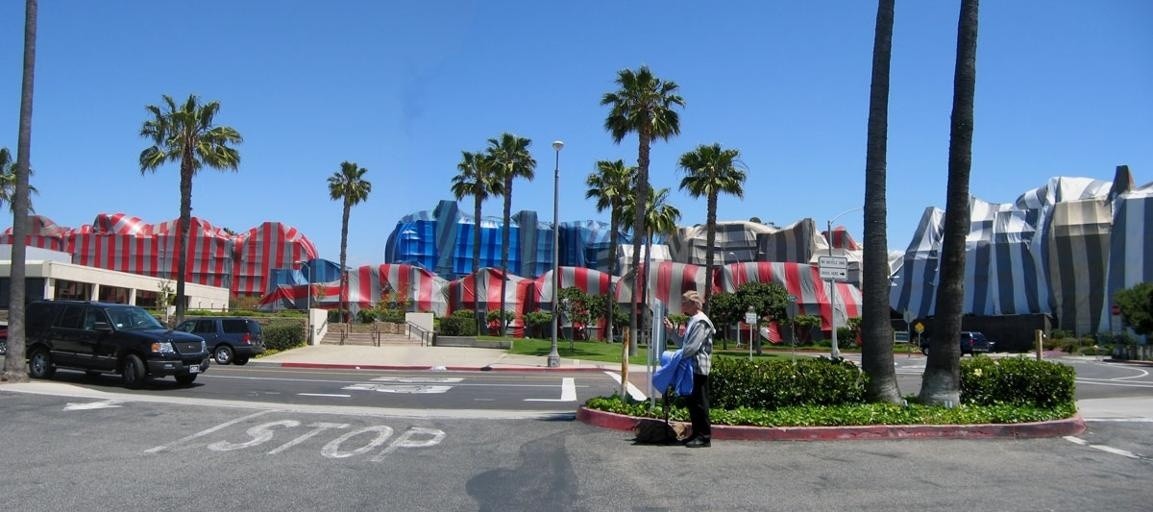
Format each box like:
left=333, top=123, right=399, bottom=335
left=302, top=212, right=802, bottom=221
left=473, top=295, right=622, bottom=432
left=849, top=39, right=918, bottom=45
left=664, top=290, right=716, bottom=448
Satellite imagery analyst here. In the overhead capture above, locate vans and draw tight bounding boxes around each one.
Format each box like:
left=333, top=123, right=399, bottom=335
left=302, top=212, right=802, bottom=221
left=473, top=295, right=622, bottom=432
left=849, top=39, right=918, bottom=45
left=919, top=331, right=990, bottom=359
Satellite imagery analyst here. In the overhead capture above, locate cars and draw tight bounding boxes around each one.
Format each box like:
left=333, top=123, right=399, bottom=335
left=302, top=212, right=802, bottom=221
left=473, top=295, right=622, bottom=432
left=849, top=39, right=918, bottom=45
left=0, top=324, right=7, bottom=356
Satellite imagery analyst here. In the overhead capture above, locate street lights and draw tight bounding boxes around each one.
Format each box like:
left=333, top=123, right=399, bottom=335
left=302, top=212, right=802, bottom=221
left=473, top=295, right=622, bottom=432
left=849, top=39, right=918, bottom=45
left=727, top=250, right=741, bottom=346
left=292, top=260, right=311, bottom=345
left=545, top=137, right=562, bottom=369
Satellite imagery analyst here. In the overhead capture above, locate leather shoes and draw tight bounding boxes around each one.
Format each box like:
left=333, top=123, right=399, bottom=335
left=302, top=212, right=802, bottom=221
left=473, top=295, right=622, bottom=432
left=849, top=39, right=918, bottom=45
left=681, top=435, right=711, bottom=448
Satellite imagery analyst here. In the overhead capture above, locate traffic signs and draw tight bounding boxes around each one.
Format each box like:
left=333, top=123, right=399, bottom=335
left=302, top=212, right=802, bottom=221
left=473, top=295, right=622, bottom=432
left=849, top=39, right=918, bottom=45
left=817, top=255, right=847, bottom=269
left=818, top=269, right=847, bottom=278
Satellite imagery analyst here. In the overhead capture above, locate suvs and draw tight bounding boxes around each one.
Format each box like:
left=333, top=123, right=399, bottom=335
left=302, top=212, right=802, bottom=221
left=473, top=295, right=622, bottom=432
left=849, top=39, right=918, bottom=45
left=172, top=315, right=267, bottom=367
left=24, top=297, right=213, bottom=392
